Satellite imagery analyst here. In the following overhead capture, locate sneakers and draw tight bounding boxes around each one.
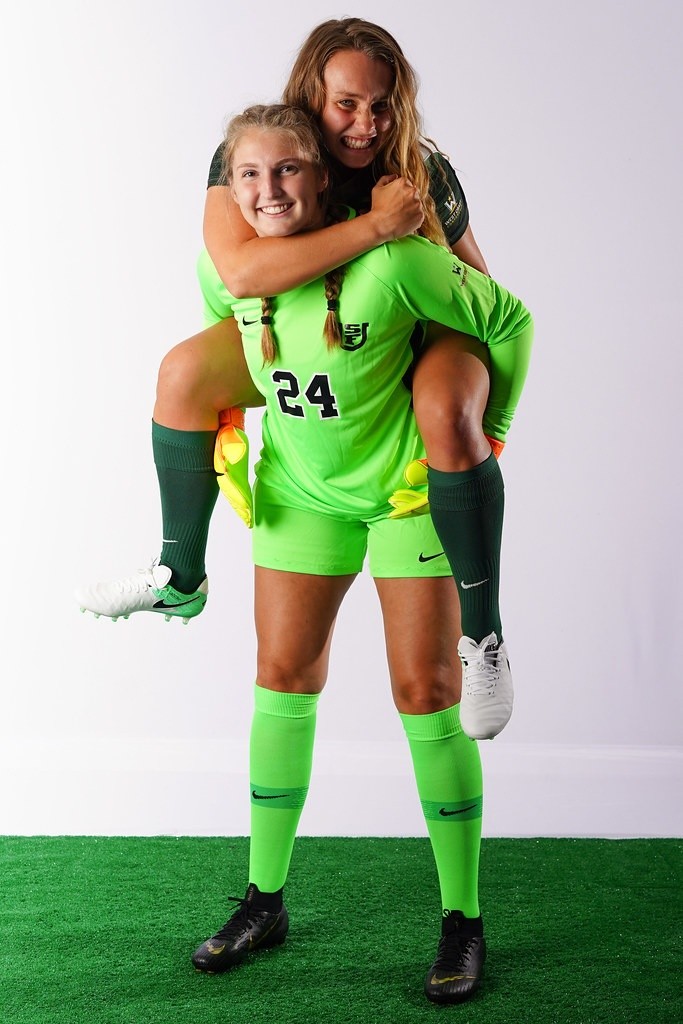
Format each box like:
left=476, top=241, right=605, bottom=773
left=458, top=633, right=512, bottom=739
left=425, top=904, right=488, bottom=1005
left=189, top=888, right=291, bottom=972
left=75, top=558, right=210, bottom=625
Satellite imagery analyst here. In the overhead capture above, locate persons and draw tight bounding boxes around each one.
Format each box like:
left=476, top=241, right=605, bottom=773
left=189, top=107, right=534, bottom=1008
left=83, top=17, right=510, bottom=740
